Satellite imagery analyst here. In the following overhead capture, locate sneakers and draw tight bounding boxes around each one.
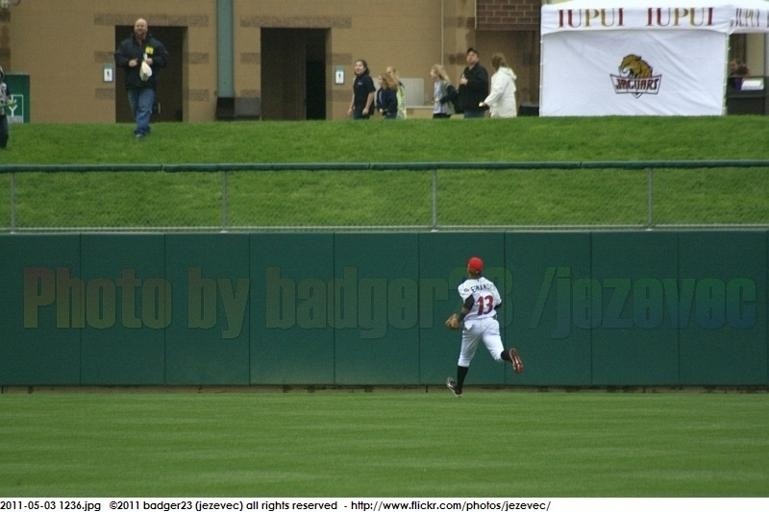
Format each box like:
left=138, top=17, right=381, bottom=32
left=509, top=348, right=524, bottom=374
left=446, top=377, right=463, bottom=398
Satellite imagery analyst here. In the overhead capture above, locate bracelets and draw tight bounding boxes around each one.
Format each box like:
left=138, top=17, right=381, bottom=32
left=456, top=312, right=465, bottom=322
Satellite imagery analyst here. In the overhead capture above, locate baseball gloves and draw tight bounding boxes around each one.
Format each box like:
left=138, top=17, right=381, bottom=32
left=445, top=313, right=464, bottom=329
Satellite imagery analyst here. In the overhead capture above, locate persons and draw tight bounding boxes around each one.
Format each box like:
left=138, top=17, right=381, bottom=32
left=377, top=73, right=397, bottom=119
left=0, top=67, right=11, bottom=148
left=458, top=48, right=489, bottom=118
left=728, top=58, right=750, bottom=90
left=430, top=64, right=457, bottom=119
left=115, top=19, right=168, bottom=139
left=445, top=256, right=524, bottom=397
left=478, top=53, right=518, bottom=119
left=347, top=60, right=375, bottom=120
left=386, top=67, right=406, bottom=119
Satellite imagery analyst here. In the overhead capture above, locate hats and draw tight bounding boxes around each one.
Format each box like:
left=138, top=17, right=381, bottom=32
left=468, top=258, right=483, bottom=275
